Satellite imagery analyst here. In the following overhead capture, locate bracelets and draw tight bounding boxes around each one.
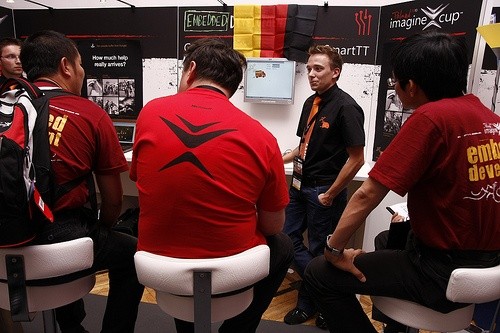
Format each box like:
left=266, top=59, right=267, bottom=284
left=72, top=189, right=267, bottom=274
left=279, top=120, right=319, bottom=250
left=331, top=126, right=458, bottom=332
left=325, top=234, right=345, bottom=255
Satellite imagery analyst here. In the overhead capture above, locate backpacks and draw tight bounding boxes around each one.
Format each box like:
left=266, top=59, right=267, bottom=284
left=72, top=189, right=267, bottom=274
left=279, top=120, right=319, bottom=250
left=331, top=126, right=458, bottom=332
left=1, top=76, right=93, bottom=248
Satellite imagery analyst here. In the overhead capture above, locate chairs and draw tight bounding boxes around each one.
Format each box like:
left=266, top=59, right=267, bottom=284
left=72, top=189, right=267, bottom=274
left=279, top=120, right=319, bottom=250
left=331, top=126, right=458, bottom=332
left=133, top=245, right=270, bottom=332
left=0, top=236, right=96, bottom=332
left=367, top=266, right=500, bottom=333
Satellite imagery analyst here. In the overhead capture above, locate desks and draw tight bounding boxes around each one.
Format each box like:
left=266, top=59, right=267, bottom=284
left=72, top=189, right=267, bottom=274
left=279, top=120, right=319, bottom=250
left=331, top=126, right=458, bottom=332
left=95, top=151, right=407, bottom=254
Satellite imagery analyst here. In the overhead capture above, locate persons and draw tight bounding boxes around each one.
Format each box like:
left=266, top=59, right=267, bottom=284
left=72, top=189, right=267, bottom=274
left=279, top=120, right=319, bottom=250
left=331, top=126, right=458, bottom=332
left=129, top=39, right=295, bottom=333
left=303, top=31, right=500, bottom=333
left=282, top=45, right=366, bottom=328
left=0, top=36, right=27, bottom=94
left=0, top=29, right=146, bottom=333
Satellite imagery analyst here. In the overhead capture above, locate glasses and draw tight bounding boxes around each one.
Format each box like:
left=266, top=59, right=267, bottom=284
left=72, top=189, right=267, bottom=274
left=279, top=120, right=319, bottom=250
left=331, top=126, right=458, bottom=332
left=0, top=55, right=19, bottom=60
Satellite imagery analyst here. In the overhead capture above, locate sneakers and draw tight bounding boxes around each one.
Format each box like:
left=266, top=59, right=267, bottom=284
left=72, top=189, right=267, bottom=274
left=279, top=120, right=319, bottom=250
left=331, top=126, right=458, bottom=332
left=283, top=306, right=315, bottom=325
left=316, top=309, right=328, bottom=330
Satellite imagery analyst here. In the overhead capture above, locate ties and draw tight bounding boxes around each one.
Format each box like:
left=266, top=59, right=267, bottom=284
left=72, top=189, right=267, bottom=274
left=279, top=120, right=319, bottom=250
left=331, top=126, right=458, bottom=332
left=299, top=97, right=321, bottom=161
